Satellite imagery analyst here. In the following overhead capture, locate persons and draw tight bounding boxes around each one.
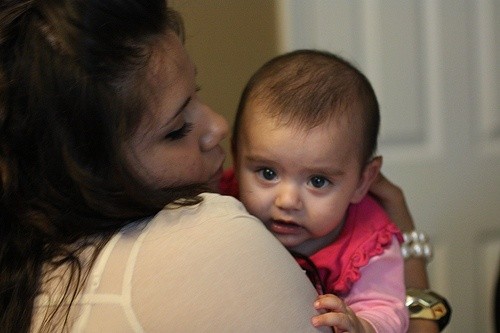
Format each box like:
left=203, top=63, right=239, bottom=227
left=0, top=0, right=453, bottom=333
left=218, top=49, right=410, bottom=333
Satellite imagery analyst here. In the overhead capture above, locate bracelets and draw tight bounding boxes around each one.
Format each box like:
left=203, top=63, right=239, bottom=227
left=401, top=231, right=435, bottom=262
left=405, top=288, right=452, bottom=331
